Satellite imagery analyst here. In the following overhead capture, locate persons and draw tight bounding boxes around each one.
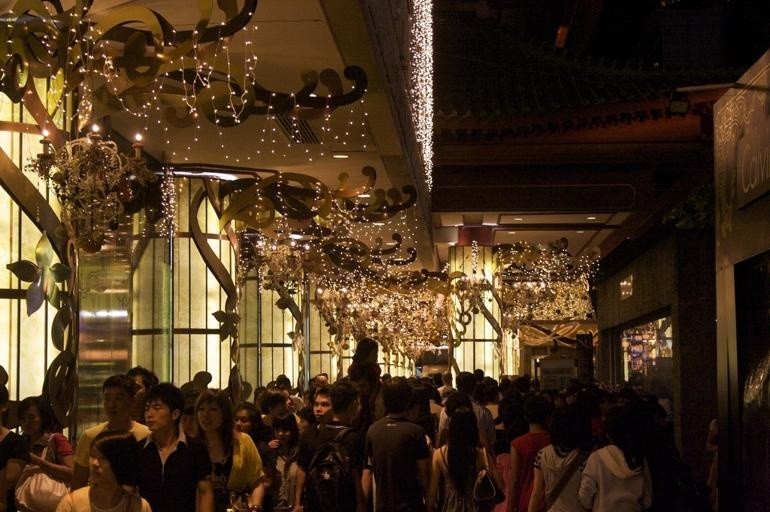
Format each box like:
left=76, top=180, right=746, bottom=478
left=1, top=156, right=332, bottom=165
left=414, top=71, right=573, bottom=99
left=2, top=336, right=720, bottom=512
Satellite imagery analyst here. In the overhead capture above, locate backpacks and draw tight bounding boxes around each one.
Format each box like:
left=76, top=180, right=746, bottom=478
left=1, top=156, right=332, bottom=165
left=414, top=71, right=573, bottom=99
left=301, top=428, right=358, bottom=511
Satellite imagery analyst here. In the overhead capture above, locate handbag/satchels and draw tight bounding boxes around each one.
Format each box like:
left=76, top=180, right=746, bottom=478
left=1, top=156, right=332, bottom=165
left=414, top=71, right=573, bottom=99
left=14, top=434, right=73, bottom=512
left=214, top=486, right=274, bottom=512
left=472, top=448, right=505, bottom=504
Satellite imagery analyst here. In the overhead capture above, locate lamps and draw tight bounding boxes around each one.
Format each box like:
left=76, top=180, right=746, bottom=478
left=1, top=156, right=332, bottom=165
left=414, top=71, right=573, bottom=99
left=3, top=3, right=375, bottom=253
